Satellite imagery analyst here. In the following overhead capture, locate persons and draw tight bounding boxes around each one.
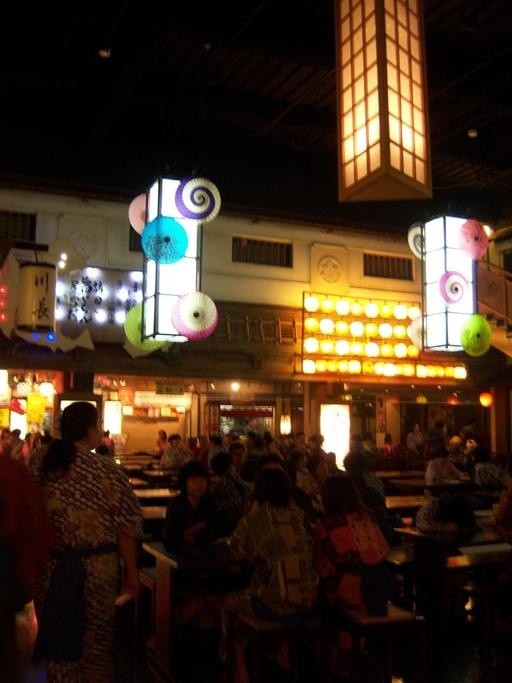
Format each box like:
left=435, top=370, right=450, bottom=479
left=3, top=402, right=142, bottom=681
left=154, top=428, right=404, bottom=681
left=405, top=420, right=511, bottom=632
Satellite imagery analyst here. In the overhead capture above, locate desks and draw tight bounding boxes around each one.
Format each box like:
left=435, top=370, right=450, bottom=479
left=107, top=446, right=512, bottom=683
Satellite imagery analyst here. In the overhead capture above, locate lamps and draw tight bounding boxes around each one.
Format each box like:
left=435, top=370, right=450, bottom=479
left=420, top=220, right=480, bottom=355
left=339, top=1, right=433, bottom=207
left=140, top=179, right=203, bottom=349
